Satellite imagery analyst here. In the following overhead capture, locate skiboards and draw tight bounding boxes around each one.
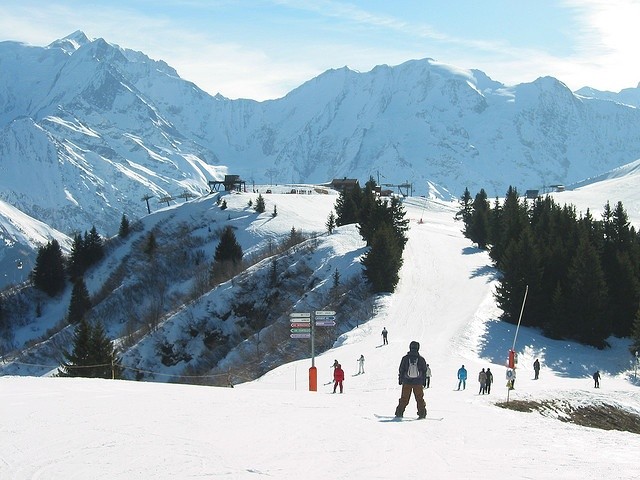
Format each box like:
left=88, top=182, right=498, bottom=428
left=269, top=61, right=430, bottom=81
left=373, top=414, right=443, bottom=422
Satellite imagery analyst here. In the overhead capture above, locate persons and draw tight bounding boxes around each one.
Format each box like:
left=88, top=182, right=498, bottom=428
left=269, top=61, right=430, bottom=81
left=333, top=365, right=346, bottom=392
left=379, top=327, right=389, bottom=344
left=331, top=359, right=339, bottom=368
left=510, top=367, right=514, bottom=388
left=534, top=358, right=540, bottom=379
left=425, top=364, right=433, bottom=386
left=478, top=369, right=485, bottom=391
left=593, top=370, right=600, bottom=387
left=458, top=364, right=468, bottom=389
left=395, top=340, right=427, bottom=419
left=486, top=368, right=493, bottom=393
left=357, top=354, right=367, bottom=372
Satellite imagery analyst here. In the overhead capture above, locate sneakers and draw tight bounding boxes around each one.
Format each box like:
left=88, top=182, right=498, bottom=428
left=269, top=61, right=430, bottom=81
left=395, top=414, right=403, bottom=417
left=418, top=415, right=425, bottom=418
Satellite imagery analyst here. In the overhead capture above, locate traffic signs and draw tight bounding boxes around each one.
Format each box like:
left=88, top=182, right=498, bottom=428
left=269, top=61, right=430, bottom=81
left=288, top=312, right=310, bottom=317
left=290, top=322, right=311, bottom=328
left=315, top=311, right=337, bottom=315
left=289, top=334, right=310, bottom=338
left=314, top=321, right=337, bottom=327
left=290, top=328, right=311, bottom=333
left=289, top=318, right=310, bottom=322
left=314, top=316, right=336, bottom=321
